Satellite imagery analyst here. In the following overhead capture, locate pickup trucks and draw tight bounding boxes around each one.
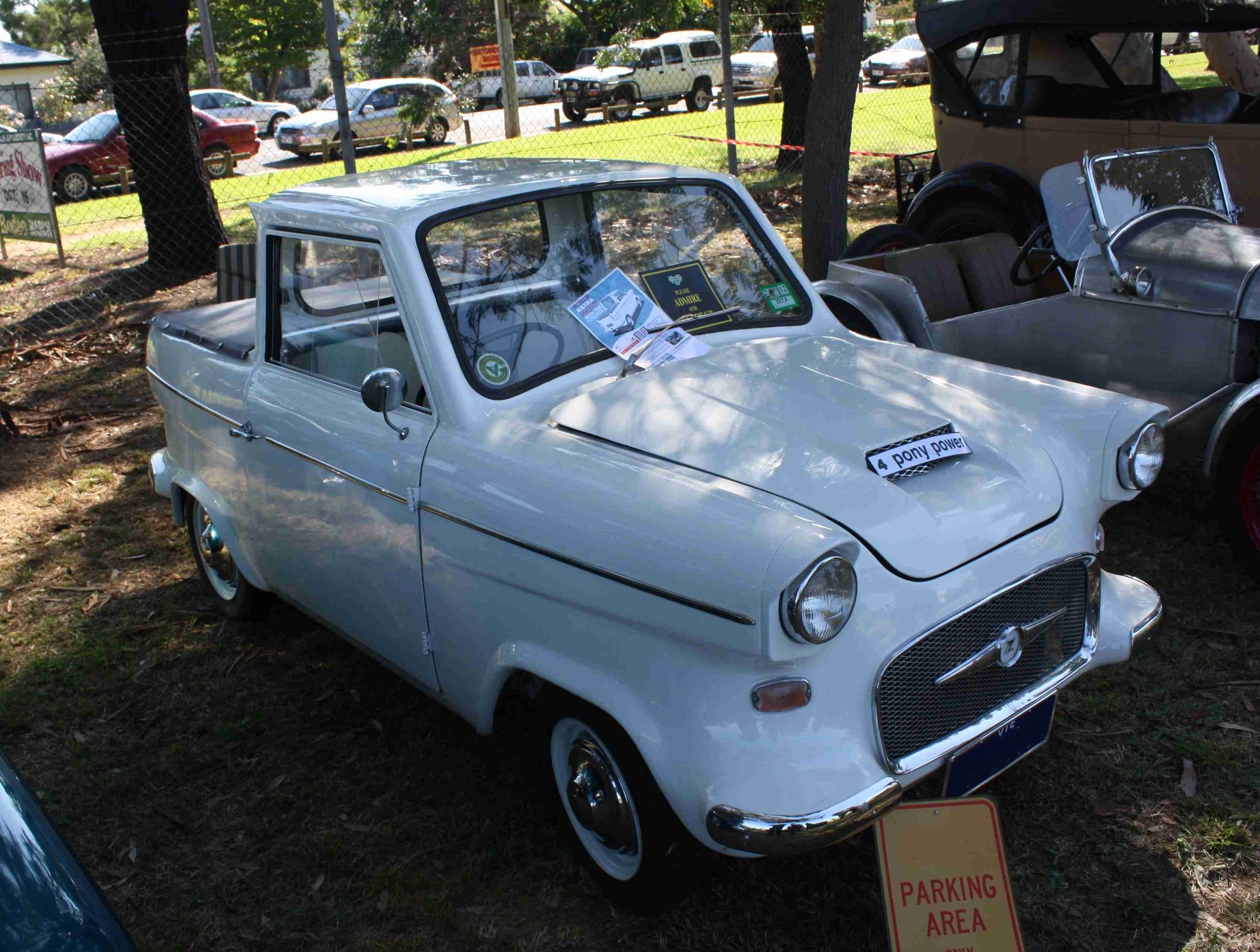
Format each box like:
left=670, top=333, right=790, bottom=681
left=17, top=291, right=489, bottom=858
left=146, top=157, right=1171, bottom=891
left=450, top=59, right=562, bottom=113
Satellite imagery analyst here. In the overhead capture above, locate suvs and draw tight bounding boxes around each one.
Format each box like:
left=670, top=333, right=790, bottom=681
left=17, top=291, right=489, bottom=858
left=730, top=25, right=826, bottom=101
left=552, top=30, right=726, bottom=122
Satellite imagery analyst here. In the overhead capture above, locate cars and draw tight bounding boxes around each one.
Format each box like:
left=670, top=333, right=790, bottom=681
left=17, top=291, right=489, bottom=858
left=42, top=109, right=263, bottom=203
left=896, top=1, right=1260, bottom=245
left=0, top=125, right=63, bottom=147
left=1159, top=29, right=1205, bottom=55
left=810, top=136, right=1260, bottom=485
left=272, top=77, right=464, bottom=160
left=861, top=32, right=985, bottom=87
left=0, top=760, right=120, bottom=952
left=185, top=89, right=301, bottom=136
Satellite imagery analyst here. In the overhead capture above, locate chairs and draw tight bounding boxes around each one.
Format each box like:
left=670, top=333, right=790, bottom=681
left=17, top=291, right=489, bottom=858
left=506, top=298, right=601, bottom=383
left=311, top=330, right=423, bottom=405
left=1165, top=85, right=1242, bottom=124
left=997, top=73, right=1068, bottom=115
left=882, top=231, right=1041, bottom=322
